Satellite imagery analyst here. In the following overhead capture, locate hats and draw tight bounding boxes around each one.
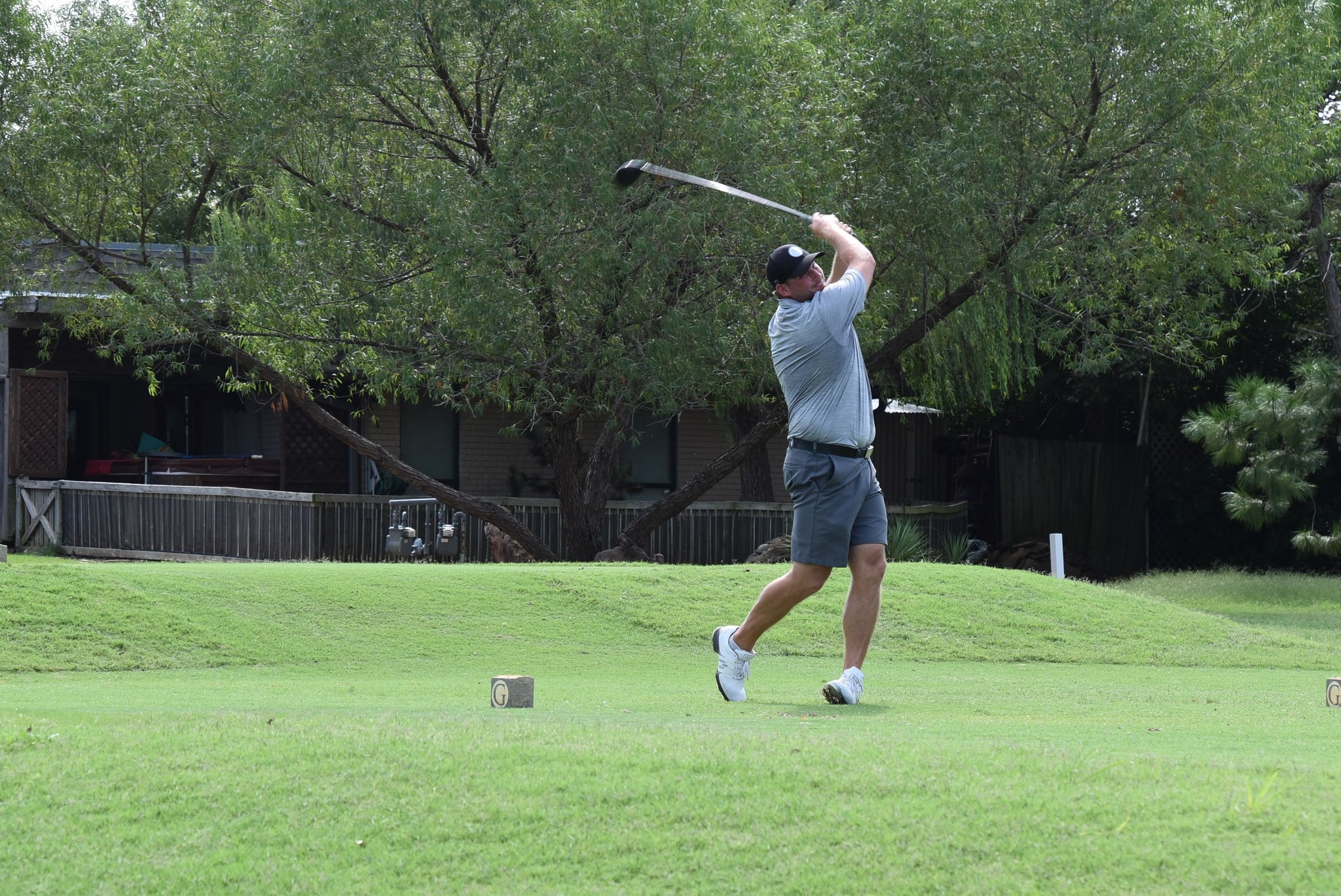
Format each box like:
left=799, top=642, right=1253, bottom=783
left=765, top=244, right=826, bottom=290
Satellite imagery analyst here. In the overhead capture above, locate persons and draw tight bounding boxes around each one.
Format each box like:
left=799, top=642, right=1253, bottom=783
left=712, top=213, right=887, bottom=702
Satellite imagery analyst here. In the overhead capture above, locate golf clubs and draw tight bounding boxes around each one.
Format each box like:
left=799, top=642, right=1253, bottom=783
left=611, top=159, right=812, bottom=222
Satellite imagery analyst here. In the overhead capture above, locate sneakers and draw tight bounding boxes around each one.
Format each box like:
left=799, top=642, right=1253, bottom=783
left=712, top=625, right=757, bottom=702
left=822, top=666, right=864, bottom=704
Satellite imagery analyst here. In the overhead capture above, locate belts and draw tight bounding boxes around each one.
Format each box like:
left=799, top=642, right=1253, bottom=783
left=791, top=439, right=874, bottom=459
